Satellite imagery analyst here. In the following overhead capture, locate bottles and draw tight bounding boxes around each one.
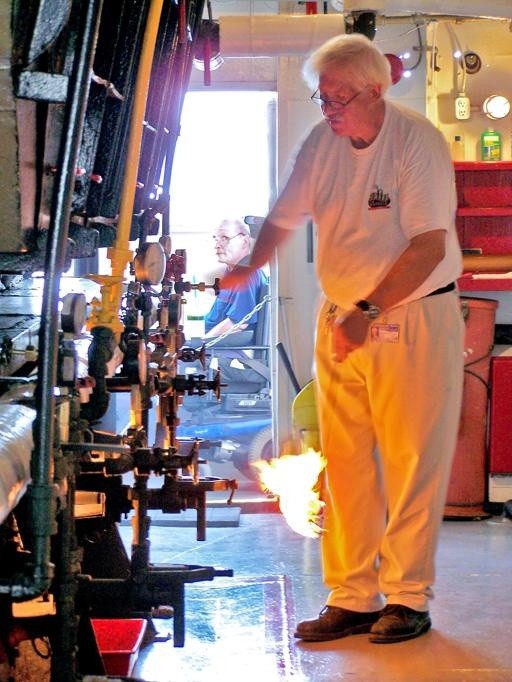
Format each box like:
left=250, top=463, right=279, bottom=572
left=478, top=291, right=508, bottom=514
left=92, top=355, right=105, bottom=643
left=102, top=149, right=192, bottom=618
left=452, top=135, right=464, bottom=162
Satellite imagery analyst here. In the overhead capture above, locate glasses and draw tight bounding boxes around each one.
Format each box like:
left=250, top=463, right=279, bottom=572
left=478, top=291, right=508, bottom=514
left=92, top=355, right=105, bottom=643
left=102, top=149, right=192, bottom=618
left=310, top=92, right=360, bottom=111
left=214, top=235, right=236, bottom=245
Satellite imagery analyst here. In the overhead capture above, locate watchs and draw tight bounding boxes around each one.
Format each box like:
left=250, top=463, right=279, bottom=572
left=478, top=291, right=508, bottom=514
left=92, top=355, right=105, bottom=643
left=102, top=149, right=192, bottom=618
left=354, top=299, right=381, bottom=320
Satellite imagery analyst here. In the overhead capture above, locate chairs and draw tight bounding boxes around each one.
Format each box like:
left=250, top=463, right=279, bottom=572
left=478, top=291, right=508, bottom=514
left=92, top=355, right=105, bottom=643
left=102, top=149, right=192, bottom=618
left=184, top=274, right=270, bottom=393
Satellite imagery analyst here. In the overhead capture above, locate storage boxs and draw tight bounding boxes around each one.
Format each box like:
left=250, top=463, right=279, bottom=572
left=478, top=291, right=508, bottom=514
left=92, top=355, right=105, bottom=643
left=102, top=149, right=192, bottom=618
left=86, top=618, right=147, bottom=679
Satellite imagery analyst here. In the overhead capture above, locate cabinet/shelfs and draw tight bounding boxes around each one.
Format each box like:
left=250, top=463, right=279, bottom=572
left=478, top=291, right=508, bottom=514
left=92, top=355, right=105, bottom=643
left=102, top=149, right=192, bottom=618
left=487, top=344, right=512, bottom=525
left=452, top=161, right=512, bottom=291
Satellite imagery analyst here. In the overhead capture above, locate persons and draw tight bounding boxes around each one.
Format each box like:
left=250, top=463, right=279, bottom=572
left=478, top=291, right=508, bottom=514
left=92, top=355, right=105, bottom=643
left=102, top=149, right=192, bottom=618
left=217, top=33, right=465, bottom=644
left=201, top=220, right=267, bottom=339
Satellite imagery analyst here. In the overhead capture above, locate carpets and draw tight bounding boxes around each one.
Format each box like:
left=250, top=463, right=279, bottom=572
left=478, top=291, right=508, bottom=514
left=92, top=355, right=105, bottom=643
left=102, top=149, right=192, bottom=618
left=120, top=575, right=300, bottom=682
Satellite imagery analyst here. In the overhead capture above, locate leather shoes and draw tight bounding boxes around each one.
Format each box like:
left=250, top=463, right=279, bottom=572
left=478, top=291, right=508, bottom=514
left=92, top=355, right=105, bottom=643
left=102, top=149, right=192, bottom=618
left=294, top=604, right=431, bottom=644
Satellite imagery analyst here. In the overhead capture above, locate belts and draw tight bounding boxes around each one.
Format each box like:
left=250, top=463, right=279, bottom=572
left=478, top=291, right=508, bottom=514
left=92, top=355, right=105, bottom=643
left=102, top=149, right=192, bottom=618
left=423, top=282, right=458, bottom=299
